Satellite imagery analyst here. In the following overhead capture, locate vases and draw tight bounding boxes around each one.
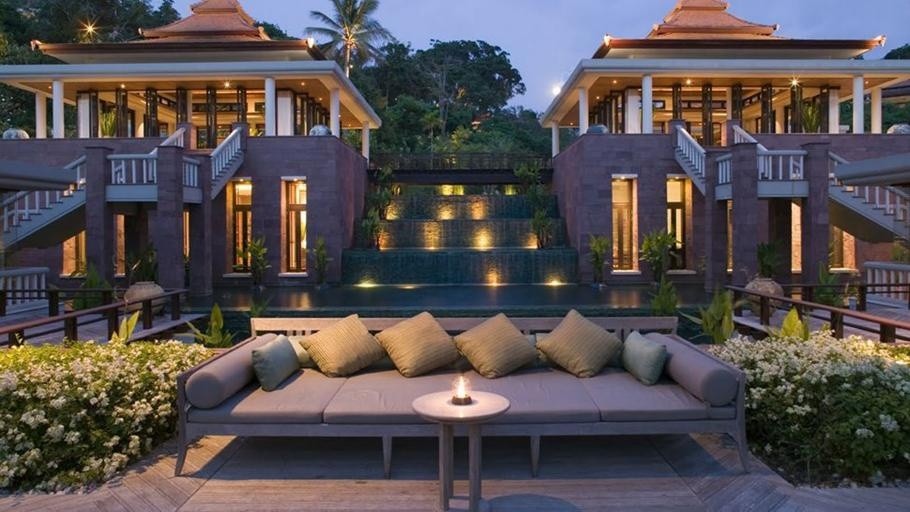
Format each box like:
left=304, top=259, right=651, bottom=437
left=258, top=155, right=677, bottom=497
left=307, top=124, right=333, bottom=138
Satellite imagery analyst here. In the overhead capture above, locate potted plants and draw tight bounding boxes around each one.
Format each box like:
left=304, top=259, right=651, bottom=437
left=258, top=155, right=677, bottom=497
left=636, top=227, right=687, bottom=299
left=2, top=110, right=34, bottom=140
left=744, top=239, right=787, bottom=319
left=120, top=243, right=168, bottom=321
left=312, top=237, right=335, bottom=291
left=101, top=111, right=117, bottom=139
left=229, top=235, right=274, bottom=298
left=586, top=233, right=610, bottom=289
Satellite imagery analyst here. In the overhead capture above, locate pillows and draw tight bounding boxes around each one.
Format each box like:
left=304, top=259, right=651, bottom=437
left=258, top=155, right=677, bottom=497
left=620, top=329, right=670, bottom=388
left=373, top=309, right=464, bottom=377
left=451, top=310, right=542, bottom=380
left=533, top=307, right=624, bottom=380
left=249, top=332, right=302, bottom=392
left=299, top=312, right=387, bottom=377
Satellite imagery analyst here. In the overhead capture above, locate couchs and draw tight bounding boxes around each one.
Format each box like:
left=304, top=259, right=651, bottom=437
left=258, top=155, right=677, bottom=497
left=173, top=314, right=753, bottom=477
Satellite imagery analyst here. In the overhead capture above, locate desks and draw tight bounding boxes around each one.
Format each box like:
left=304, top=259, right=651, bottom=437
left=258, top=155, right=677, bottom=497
left=410, top=389, right=512, bottom=511
